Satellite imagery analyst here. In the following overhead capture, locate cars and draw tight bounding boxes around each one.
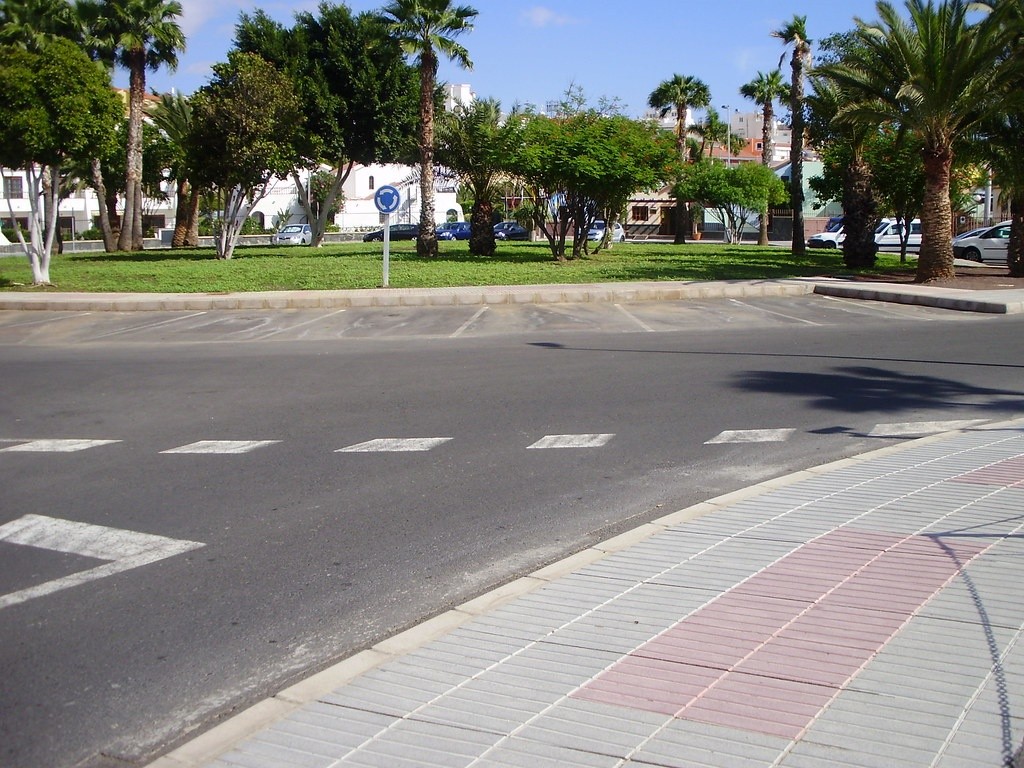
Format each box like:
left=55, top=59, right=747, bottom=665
left=577, top=220, right=625, bottom=243
left=272, top=224, right=312, bottom=246
left=493, top=222, right=531, bottom=241
left=949, top=227, right=1011, bottom=257
left=952, top=218, right=1013, bottom=263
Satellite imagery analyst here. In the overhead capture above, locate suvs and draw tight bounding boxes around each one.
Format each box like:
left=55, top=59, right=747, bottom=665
left=362, top=224, right=418, bottom=242
left=871, top=215, right=922, bottom=252
left=808, top=223, right=847, bottom=250
left=824, top=217, right=841, bottom=231
left=435, top=222, right=471, bottom=240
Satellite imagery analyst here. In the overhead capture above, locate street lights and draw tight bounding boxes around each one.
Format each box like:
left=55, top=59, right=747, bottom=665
left=721, top=105, right=730, bottom=169
